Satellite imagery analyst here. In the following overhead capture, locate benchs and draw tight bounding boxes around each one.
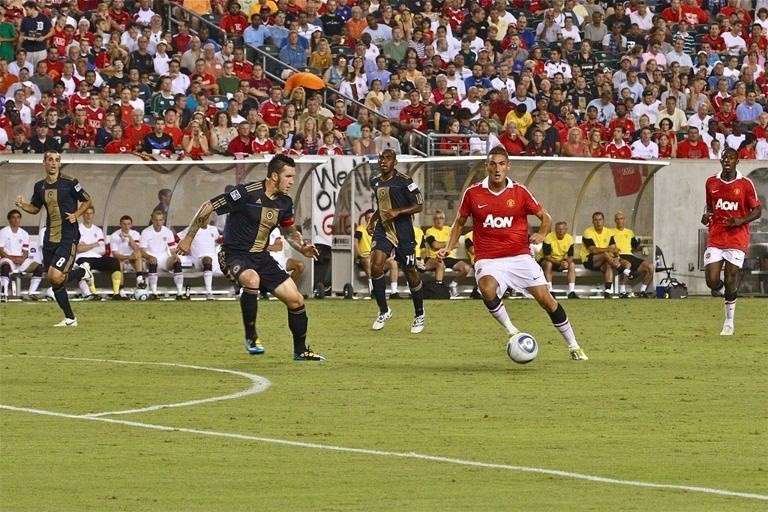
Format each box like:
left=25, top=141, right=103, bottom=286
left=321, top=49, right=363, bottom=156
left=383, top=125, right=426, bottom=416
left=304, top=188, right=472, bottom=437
left=0, top=263, right=273, bottom=299
left=358, top=236, right=633, bottom=297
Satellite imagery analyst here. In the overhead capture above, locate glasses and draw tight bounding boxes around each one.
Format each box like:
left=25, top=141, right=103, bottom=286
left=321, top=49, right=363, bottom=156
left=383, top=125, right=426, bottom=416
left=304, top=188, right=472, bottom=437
left=533, top=114, right=540, bottom=117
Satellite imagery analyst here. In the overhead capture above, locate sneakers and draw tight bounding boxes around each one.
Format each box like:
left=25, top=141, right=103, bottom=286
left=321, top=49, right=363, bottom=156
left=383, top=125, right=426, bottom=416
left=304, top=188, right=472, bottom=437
left=409, top=309, right=426, bottom=334
left=368, top=286, right=652, bottom=300
left=135, top=281, right=148, bottom=290
left=570, top=349, right=588, bottom=361
left=372, top=307, right=393, bottom=331
left=78, top=262, right=94, bottom=286
left=628, top=271, right=645, bottom=282
left=51, top=317, right=79, bottom=327
left=244, top=337, right=265, bottom=354
left=721, top=321, right=735, bottom=337
left=294, top=351, right=328, bottom=362
left=2, top=291, right=270, bottom=302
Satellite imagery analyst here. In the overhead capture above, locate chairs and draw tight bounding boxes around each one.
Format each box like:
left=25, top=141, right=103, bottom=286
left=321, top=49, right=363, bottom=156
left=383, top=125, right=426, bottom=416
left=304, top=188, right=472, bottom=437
left=1, top=1, right=677, bottom=159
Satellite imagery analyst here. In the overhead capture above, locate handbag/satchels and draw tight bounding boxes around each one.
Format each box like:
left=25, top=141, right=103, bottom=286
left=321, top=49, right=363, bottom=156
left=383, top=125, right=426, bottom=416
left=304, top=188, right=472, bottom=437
left=419, top=280, right=451, bottom=299
left=664, top=280, right=688, bottom=299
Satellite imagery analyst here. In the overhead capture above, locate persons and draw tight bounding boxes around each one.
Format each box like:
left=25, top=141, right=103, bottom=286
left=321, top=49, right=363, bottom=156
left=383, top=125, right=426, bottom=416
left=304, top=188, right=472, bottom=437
left=365, top=148, right=427, bottom=335
left=699, top=147, right=761, bottom=337
left=434, top=145, right=587, bottom=361
left=176, top=153, right=325, bottom=363
left=14, top=149, right=93, bottom=327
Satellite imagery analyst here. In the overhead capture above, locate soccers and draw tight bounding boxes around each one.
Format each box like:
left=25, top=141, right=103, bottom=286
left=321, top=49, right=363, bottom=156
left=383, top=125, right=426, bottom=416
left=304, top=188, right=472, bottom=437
left=506, top=333, right=538, bottom=364
left=135, top=288, right=150, bottom=302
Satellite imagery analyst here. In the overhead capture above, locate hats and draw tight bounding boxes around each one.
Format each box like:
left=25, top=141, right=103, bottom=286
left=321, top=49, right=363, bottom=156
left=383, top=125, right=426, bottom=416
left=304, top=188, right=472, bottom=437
left=507, top=43, right=518, bottom=50
left=38, top=119, right=50, bottom=127
